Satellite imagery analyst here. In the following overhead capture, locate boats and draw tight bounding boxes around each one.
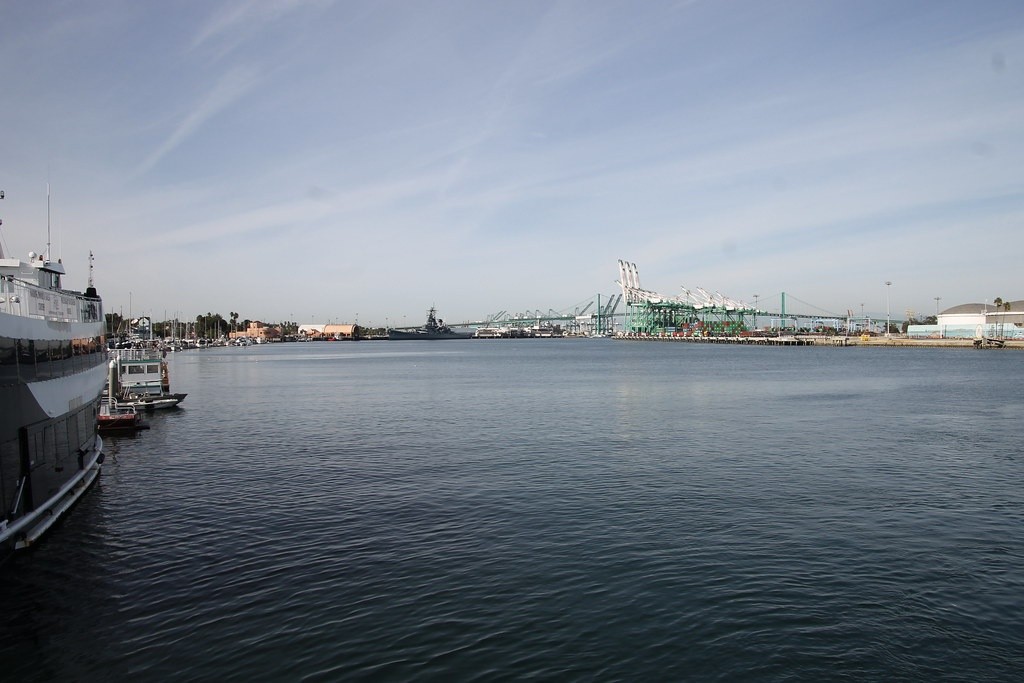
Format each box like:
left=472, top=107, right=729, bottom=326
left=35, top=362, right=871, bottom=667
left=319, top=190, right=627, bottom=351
left=974, top=336, right=1005, bottom=349
left=0, top=183, right=112, bottom=566
left=388, top=302, right=475, bottom=340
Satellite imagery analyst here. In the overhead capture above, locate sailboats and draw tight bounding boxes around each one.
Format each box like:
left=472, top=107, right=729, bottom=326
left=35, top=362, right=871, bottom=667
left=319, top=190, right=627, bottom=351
left=98, top=291, right=266, bottom=431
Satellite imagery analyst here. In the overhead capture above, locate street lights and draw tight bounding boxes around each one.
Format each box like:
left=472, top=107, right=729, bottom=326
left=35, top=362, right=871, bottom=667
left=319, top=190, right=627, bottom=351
left=885, top=281, right=892, bottom=335
left=861, top=304, right=864, bottom=330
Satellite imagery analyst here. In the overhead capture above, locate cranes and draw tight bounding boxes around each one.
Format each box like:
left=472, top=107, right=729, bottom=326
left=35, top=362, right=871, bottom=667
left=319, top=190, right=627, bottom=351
left=615, top=259, right=767, bottom=336
